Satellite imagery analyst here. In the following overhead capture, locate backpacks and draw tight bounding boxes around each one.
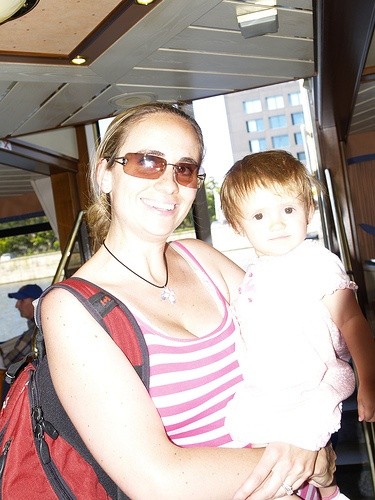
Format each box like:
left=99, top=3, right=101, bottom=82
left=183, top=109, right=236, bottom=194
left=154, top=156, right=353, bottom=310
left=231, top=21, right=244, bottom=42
left=0, top=277, right=151, bottom=499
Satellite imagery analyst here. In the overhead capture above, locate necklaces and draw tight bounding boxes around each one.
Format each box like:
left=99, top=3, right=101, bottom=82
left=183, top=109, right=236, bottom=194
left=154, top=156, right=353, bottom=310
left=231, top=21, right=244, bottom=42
left=102, top=240, right=176, bottom=303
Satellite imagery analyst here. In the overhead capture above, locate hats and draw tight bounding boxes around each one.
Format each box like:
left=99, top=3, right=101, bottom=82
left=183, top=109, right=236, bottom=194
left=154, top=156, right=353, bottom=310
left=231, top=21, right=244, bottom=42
left=8, top=284, right=43, bottom=299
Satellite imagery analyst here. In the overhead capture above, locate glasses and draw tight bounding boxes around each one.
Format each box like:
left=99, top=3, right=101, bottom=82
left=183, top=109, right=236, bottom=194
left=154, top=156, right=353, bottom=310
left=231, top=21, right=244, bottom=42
left=104, top=153, right=206, bottom=189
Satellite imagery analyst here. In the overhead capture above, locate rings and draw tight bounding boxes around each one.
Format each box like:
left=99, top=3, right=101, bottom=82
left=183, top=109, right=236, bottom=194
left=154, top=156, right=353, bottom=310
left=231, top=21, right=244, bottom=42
left=283, top=484, right=293, bottom=496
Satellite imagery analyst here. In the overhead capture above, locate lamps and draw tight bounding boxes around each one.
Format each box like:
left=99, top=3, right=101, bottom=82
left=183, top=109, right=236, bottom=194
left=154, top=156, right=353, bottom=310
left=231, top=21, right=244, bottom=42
left=235, top=2, right=278, bottom=29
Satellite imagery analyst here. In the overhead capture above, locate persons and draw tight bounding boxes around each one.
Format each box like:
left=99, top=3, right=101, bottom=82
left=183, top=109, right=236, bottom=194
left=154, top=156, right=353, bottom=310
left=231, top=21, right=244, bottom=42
left=33, top=101, right=336, bottom=500
left=0, top=284, right=43, bottom=369
left=218, top=150, right=375, bottom=500
